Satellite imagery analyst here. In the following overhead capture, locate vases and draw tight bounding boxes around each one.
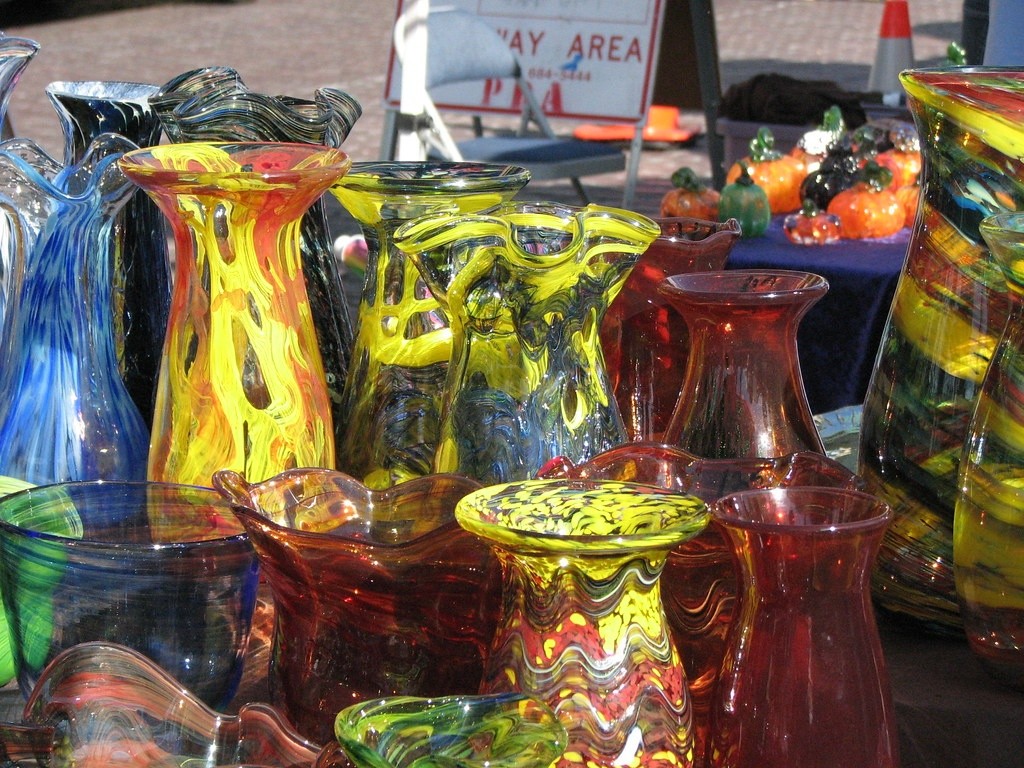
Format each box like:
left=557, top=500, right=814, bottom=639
left=0, top=25, right=1024, bottom=768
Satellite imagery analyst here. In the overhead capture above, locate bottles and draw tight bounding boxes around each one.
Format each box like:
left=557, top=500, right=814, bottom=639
left=0, top=36, right=1024, bottom=768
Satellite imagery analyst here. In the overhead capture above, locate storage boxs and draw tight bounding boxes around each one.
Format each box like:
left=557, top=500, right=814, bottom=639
left=716, top=104, right=911, bottom=185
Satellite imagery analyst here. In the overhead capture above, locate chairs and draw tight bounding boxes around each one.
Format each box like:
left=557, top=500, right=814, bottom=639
left=392, top=6, right=628, bottom=204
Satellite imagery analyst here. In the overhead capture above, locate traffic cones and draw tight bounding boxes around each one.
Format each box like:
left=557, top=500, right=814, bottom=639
left=868, top=0, right=916, bottom=95
